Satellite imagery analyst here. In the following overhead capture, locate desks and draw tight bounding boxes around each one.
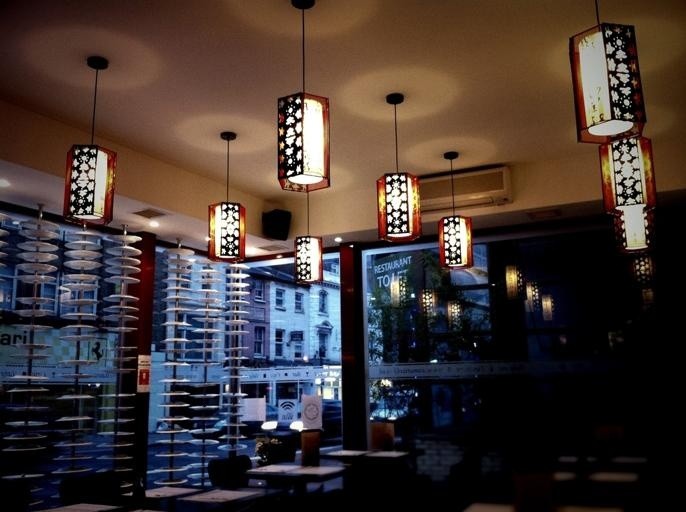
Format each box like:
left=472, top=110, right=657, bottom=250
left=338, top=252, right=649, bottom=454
left=450, top=455, right=652, bottom=512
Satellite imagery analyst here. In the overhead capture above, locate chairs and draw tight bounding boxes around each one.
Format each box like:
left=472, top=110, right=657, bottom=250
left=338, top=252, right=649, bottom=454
left=207, top=455, right=253, bottom=488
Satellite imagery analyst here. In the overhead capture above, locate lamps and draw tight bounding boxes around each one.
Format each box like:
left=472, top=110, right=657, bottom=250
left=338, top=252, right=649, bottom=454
left=390, top=256, right=655, bottom=331
left=567, top=0, right=647, bottom=146
left=597, top=135, right=653, bottom=213
left=614, top=208, right=653, bottom=253
left=293, top=193, right=324, bottom=285
left=376, top=93, right=422, bottom=243
left=207, top=131, right=246, bottom=264
left=277, top=1, right=331, bottom=194
left=61, top=54, right=117, bottom=229
left=436, top=151, right=474, bottom=270
